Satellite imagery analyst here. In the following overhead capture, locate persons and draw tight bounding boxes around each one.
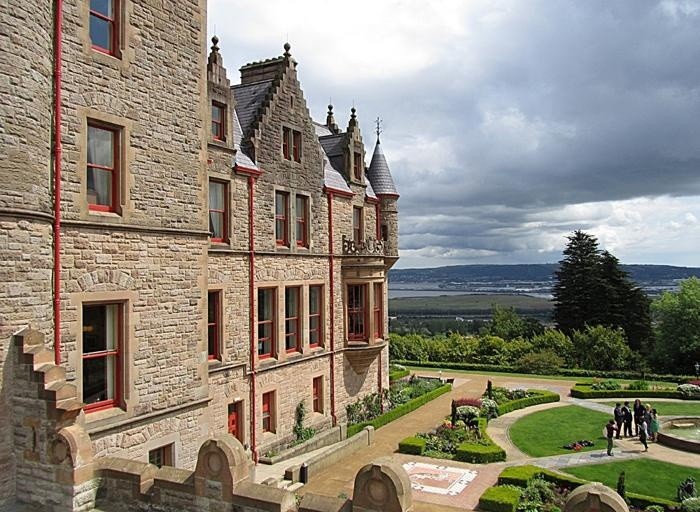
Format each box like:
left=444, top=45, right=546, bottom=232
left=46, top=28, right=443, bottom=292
left=606, top=418, right=618, bottom=456
left=650, top=408, right=659, bottom=443
left=643, top=403, right=654, bottom=440
left=636, top=415, right=650, bottom=451
left=685, top=478, right=694, bottom=495
left=633, top=398, right=647, bottom=437
left=622, top=401, right=634, bottom=436
left=614, top=402, right=625, bottom=439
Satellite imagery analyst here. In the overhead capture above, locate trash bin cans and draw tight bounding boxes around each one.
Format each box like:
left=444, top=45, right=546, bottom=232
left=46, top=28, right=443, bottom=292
left=301, top=463, right=308, bottom=484
